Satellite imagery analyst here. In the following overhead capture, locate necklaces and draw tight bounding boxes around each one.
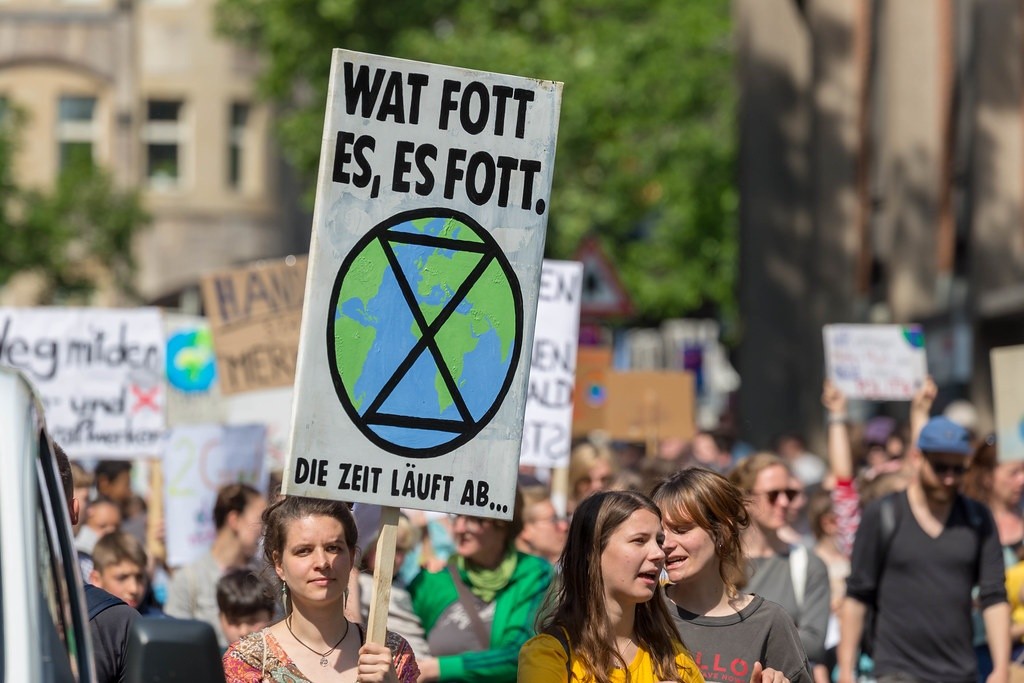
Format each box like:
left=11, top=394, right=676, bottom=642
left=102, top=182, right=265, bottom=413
left=285, top=616, right=349, bottom=667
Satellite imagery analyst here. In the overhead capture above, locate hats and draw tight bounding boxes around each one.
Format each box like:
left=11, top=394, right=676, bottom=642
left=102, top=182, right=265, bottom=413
left=918, top=416, right=969, bottom=455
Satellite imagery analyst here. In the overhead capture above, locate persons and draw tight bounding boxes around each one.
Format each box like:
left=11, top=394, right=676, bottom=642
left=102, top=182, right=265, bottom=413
left=962, top=430, right=1024, bottom=683
left=216, top=565, right=276, bottom=647
left=648, top=466, right=815, bottom=683
left=52, top=441, right=162, bottom=682
left=836, top=416, right=1013, bottom=683
left=222, top=496, right=420, bottom=683
left=365, top=476, right=561, bottom=683
left=165, top=481, right=264, bottom=651
left=725, top=450, right=830, bottom=683
left=519, top=375, right=938, bottom=557
left=518, top=491, right=707, bottom=683
left=350, top=502, right=412, bottom=582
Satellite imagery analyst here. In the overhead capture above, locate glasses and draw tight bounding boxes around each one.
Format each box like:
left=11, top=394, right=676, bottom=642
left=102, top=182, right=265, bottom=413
left=749, top=489, right=797, bottom=507
left=933, top=463, right=968, bottom=476
